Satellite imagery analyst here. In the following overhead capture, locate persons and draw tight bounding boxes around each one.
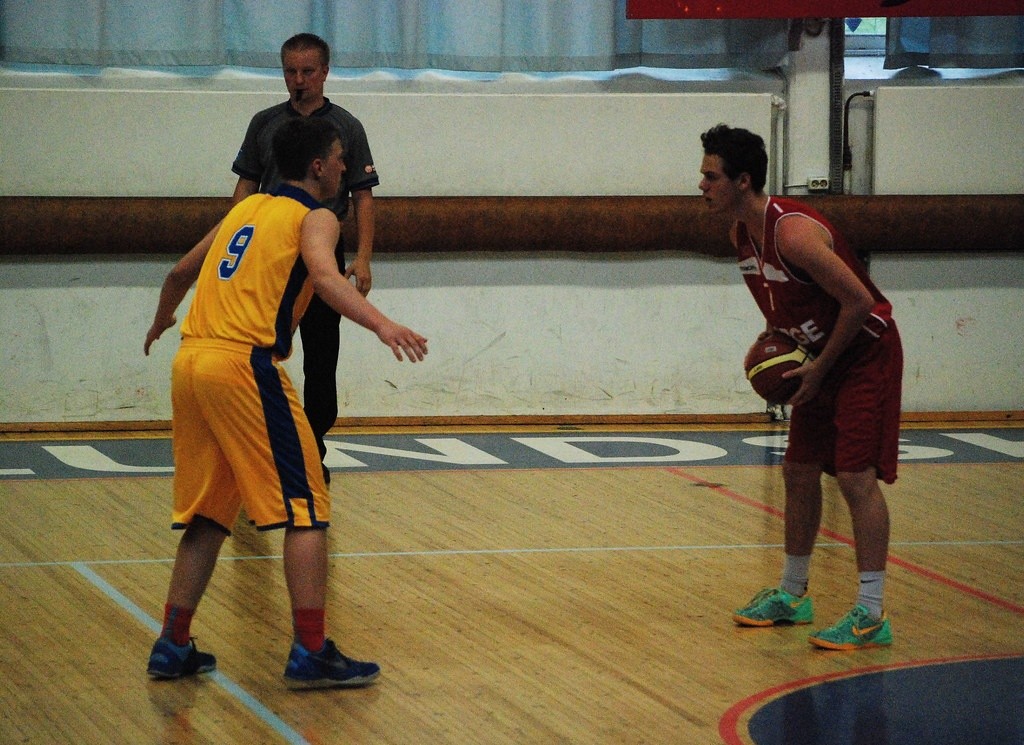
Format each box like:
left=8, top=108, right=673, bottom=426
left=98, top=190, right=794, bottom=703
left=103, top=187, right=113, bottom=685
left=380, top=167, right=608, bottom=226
left=143, top=117, right=428, bottom=692
left=697, top=123, right=904, bottom=652
left=229, top=32, right=380, bottom=490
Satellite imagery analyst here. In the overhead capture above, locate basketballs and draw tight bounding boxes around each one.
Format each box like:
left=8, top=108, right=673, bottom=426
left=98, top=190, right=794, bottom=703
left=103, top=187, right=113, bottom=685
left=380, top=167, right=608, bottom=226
left=746, top=332, right=817, bottom=406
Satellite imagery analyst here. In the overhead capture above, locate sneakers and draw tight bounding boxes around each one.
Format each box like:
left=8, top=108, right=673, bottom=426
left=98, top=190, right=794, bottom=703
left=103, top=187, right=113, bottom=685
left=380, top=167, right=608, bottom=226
left=146, top=634, right=216, bottom=677
left=806, top=604, right=894, bottom=649
left=282, top=639, right=381, bottom=688
left=731, top=586, right=815, bottom=626
